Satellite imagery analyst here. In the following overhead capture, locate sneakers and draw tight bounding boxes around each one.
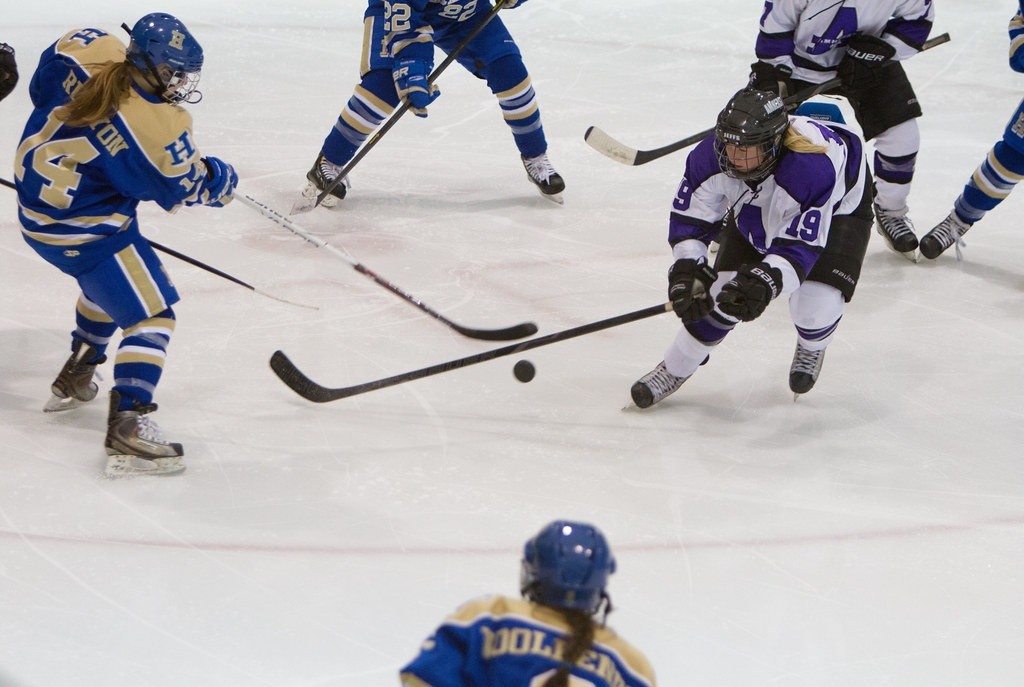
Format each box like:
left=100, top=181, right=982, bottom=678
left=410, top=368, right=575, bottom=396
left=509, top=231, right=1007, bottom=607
left=916, top=209, right=972, bottom=260
left=873, top=180, right=918, bottom=263
left=789, top=341, right=826, bottom=402
left=621, top=354, right=709, bottom=412
left=521, top=153, right=565, bottom=204
left=104, top=389, right=184, bottom=475
left=43, top=339, right=98, bottom=412
left=302, top=149, right=351, bottom=208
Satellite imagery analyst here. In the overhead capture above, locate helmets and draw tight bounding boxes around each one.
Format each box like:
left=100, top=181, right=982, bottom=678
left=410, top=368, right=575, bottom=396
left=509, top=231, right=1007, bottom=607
left=520, top=519, right=621, bottom=612
left=126, top=12, right=203, bottom=104
left=712, top=88, right=789, bottom=179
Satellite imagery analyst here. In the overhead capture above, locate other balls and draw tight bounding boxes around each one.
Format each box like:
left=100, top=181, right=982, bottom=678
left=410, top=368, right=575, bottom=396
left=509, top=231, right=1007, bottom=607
left=514, top=359, right=536, bottom=383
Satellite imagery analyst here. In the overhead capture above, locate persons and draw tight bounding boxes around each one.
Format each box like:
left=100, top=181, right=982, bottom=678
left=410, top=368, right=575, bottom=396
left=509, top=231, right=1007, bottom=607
left=747, top=0, right=934, bottom=252
left=919, top=0, right=1024, bottom=259
left=399, top=519, right=659, bottom=687
left=306, top=0, right=566, bottom=199
left=630, top=85, right=874, bottom=408
left=14, top=12, right=239, bottom=458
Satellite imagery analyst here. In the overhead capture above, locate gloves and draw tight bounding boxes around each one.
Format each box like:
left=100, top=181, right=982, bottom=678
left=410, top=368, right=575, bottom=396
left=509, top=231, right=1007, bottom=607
left=667, top=255, right=718, bottom=323
left=189, top=156, right=239, bottom=207
left=392, top=57, right=441, bottom=118
left=718, top=261, right=782, bottom=322
left=746, top=60, right=792, bottom=98
left=835, top=33, right=896, bottom=95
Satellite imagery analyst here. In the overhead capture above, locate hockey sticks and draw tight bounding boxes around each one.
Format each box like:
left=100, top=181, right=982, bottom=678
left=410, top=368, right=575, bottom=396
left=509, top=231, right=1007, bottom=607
left=0, top=177, right=321, bottom=312
left=583, top=31, right=952, bottom=167
left=268, top=291, right=710, bottom=402
left=229, top=188, right=541, bottom=341
left=290, top=0, right=506, bottom=216
